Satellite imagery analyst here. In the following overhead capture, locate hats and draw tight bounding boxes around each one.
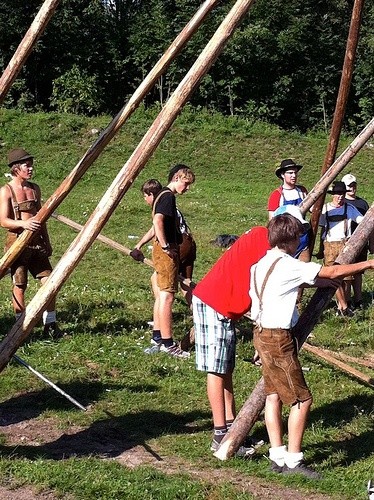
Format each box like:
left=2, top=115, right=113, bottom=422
left=168, top=164, right=189, bottom=183
left=340, top=174, right=357, bottom=186
left=327, top=181, right=352, bottom=194
left=275, top=158, right=303, bottom=179
left=7, top=148, right=34, bottom=166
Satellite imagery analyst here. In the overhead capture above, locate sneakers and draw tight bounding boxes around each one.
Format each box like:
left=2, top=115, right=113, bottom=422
left=209, top=438, right=264, bottom=457
left=150, top=337, right=192, bottom=359
left=265, top=457, right=321, bottom=480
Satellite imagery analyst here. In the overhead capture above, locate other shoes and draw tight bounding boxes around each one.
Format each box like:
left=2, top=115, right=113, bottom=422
left=337, top=308, right=355, bottom=318
left=43, top=322, right=64, bottom=339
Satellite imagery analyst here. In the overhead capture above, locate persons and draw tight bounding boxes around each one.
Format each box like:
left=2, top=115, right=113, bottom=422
left=245, top=213, right=374, bottom=480
left=2, top=147, right=65, bottom=343
left=339, top=174, right=374, bottom=310
left=269, top=159, right=314, bottom=306
left=192, top=206, right=311, bottom=457
left=150, top=165, right=196, bottom=360
left=134, top=179, right=196, bottom=328
left=314, top=182, right=366, bottom=317
